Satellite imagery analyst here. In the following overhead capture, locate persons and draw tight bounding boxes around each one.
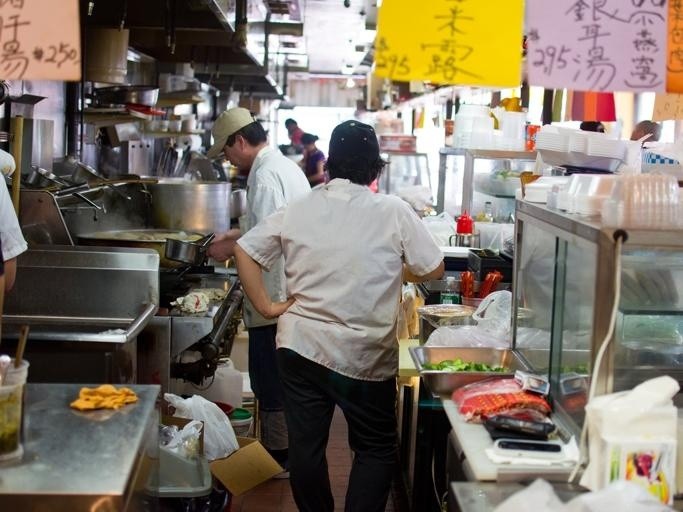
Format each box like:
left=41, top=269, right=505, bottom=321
left=628, top=118, right=662, bottom=150
left=231, top=121, right=448, bottom=510
left=299, top=133, right=327, bottom=189
left=199, top=105, right=313, bottom=482
left=0, top=171, right=29, bottom=294
left=284, top=118, right=305, bottom=152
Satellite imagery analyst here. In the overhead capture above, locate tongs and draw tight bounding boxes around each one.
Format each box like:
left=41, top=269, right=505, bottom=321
left=192, top=232, right=216, bottom=246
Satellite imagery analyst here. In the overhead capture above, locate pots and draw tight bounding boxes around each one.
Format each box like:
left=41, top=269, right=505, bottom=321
left=137, top=178, right=241, bottom=241
left=84, top=85, right=160, bottom=109
left=72, top=161, right=132, bottom=202
left=24, top=164, right=100, bottom=211
left=164, top=238, right=210, bottom=269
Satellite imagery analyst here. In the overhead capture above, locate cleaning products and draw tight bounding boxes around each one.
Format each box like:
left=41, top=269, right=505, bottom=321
left=182, top=62, right=196, bottom=80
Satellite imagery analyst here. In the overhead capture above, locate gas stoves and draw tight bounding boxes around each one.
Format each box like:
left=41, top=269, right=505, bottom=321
left=150, top=272, right=239, bottom=355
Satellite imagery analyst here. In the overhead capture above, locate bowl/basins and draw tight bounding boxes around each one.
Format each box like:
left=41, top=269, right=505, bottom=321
left=415, top=304, right=476, bottom=345
left=380, top=135, right=415, bottom=153
left=77, top=227, right=207, bottom=271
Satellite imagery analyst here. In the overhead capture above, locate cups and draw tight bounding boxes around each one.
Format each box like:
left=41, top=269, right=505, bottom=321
left=142, top=119, right=197, bottom=135
left=460, top=295, right=485, bottom=305
left=519, top=170, right=537, bottom=198
left=448, top=233, right=480, bottom=248
left=444, top=120, right=454, bottom=146
left=157, top=73, right=200, bottom=94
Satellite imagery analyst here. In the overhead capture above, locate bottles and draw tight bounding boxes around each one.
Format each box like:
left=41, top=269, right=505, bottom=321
left=456, top=209, right=473, bottom=235
left=440, top=275, right=460, bottom=306
left=482, top=201, right=493, bottom=221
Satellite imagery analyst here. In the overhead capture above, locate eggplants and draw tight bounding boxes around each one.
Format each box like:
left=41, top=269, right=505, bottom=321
left=483, top=414, right=558, bottom=441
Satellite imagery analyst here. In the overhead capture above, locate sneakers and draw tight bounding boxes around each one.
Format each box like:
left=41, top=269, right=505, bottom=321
left=272, top=462, right=290, bottom=480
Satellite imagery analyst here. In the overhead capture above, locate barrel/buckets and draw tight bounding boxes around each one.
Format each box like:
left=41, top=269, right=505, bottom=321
left=226, top=413, right=255, bottom=436
left=203, top=358, right=243, bottom=409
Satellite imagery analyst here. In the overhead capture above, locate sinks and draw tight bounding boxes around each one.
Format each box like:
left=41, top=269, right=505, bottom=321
left=0, top=311, right=135, bottom=384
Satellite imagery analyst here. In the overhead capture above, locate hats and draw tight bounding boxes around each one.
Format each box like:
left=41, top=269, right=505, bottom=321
left=207, top=107, right=258, bottom=158
left=328, top=120, right=380, bottom=171
